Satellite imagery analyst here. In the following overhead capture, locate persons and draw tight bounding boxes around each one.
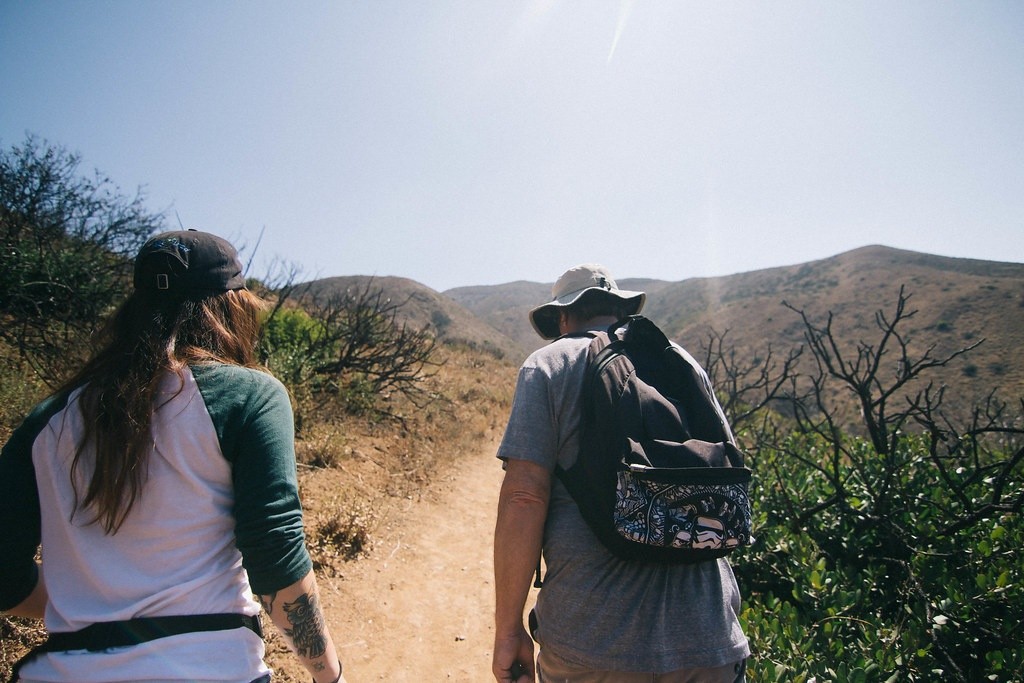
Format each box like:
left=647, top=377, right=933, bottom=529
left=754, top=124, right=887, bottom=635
left=492, top=267, right=750, bottom=683
left=0, top=229, right=347, bottom=683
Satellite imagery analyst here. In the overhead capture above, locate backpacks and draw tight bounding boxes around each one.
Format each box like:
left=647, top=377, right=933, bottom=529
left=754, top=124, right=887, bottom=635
left=565, top=314, right=750, bottom=565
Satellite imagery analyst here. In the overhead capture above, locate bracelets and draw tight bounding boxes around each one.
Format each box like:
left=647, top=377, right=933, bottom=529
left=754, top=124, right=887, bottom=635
left=313, top=660, right=342, bottom=683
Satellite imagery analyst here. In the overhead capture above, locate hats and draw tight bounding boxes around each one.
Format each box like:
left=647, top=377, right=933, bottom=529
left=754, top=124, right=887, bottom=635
left=528, top=265, right=648, bottom=342
left=132, top=230, right=243, bottom=303
